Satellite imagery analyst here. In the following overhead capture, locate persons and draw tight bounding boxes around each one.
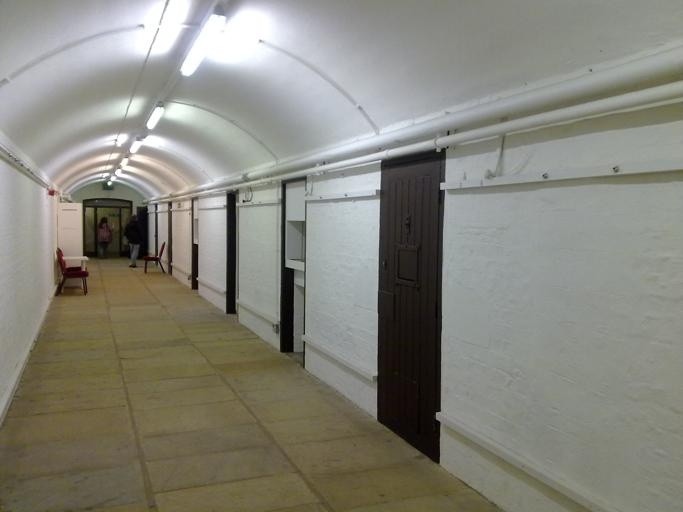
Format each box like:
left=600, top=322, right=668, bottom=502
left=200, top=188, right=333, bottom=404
left=124, top=214, right=144, bottom=268
left=97, top=216, right=114, bottom=259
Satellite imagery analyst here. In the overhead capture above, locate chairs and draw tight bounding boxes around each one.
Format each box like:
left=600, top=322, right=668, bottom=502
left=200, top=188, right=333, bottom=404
left=53, top=248, right=88, bottom=296
left=142, top=241, right=165, bottom=273
